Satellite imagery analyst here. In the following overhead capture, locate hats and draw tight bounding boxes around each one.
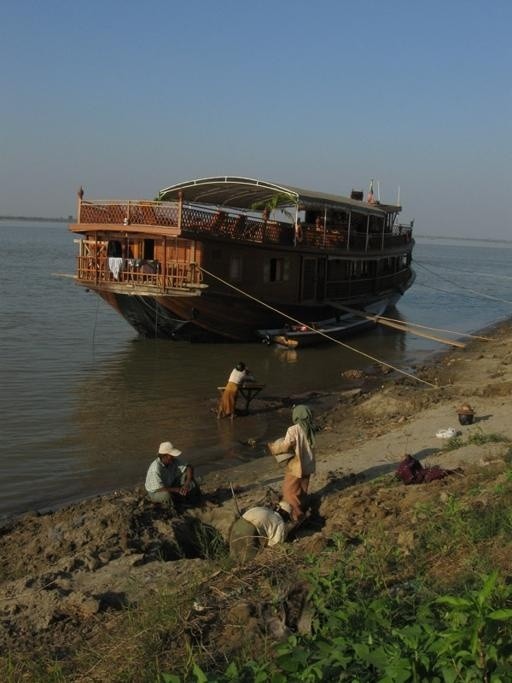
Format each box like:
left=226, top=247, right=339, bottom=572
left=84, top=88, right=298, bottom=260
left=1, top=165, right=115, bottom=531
left=159, top=441, right=182, bottom=457
left=276, top=500, right=293, bottom=520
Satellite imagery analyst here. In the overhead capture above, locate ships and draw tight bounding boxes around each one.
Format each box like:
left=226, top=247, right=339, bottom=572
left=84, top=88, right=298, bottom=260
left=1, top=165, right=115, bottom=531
left=68, top=177, right=415, bottom=344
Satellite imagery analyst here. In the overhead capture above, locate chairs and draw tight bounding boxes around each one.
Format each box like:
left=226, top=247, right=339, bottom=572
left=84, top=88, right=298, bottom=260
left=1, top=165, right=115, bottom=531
left=108, top=203, right=247, bottom=235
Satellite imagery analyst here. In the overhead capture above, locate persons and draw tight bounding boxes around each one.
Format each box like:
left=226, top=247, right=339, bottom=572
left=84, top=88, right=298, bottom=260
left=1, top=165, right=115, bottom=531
left=146, top=442, right=197, bottom=512
left=270, top=405, right=317, bottom=519
left=217, top=363, right=255, bottom=421
left=229, top=506, right=294, bottom=556
